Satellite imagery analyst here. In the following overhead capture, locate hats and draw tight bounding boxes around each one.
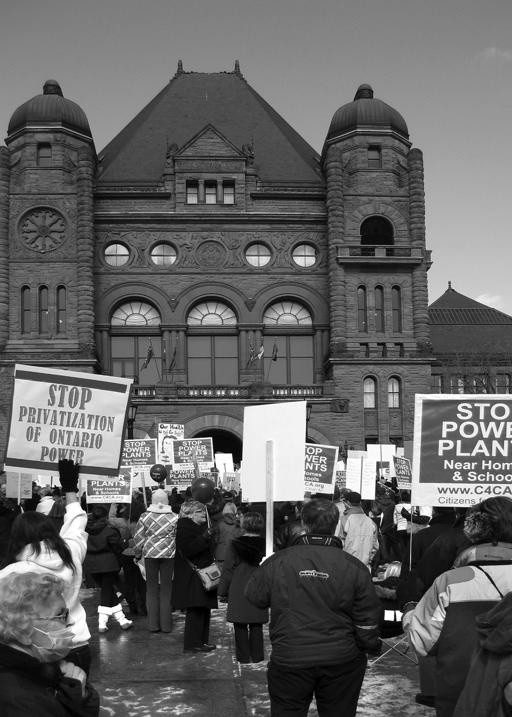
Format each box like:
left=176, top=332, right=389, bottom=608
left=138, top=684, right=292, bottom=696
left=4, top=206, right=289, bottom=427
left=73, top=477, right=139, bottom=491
left=152, top=489, right=169, bottom=505
left=345, top=492, right=361, bottom=503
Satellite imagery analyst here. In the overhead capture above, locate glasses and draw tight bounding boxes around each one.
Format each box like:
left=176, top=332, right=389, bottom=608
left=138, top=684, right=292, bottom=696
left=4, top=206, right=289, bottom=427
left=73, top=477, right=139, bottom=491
left=33, top=608, right=69, bottom=623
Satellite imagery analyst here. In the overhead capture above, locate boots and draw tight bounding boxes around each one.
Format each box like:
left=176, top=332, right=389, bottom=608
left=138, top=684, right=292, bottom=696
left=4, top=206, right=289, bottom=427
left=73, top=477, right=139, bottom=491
left=112, top=603, right=133, bottom=630
left=97, top=605, right=112, bottom=632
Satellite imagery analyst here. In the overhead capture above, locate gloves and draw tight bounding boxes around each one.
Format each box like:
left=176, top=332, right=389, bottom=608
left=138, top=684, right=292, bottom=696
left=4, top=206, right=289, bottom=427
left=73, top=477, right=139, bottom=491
left=59, top=458, right=80, bottom=493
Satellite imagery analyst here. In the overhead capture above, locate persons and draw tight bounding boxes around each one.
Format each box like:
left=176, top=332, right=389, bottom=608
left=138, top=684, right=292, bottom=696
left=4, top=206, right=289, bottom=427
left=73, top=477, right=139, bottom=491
left=397, top=494, right=511, bottom=716
left=133, top=489, right=178, bottom=633
left=0, top=560, right=98, bottom=716
left=83, top=507, right=133, bottom=632
left=171, top=478, right=218, bottom=652
left=1, top=472, right=65, bottom=566
left=6, top=459, right=92, bottom=684
left=79, top=469, right=266, bottom=615
left=218, top=512, right=268, bottom=664
left=452, top=592, right=511, bottom=717
left=273, top=496, right=312, bottom=554
left=334, top=475, right=472, bottom=624
left=245, top=497, right=382, bottom=717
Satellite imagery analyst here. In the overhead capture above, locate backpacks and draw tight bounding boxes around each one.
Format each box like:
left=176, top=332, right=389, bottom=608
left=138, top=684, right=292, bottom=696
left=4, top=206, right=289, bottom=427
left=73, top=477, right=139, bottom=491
left=198, top=563, right=222, bottom=590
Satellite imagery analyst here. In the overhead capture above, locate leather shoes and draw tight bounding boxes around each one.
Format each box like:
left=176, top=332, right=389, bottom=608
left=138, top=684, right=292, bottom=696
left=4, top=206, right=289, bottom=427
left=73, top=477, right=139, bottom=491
left=184, top=643, right=216, bottom=652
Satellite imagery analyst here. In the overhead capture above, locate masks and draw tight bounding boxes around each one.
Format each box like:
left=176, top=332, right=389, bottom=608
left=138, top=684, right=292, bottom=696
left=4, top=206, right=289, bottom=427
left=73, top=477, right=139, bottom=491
left=27, top=623, right=77, bottom=663
